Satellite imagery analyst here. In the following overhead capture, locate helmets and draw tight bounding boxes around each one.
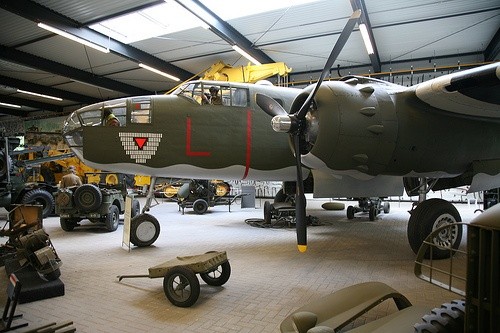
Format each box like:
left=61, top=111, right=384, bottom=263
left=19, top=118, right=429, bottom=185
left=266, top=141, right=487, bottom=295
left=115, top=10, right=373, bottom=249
left=68, top=166, right=76, bottom=173
left=104, top=109, right=112, bottom=117
left=209, top=87, right=219, bottom=95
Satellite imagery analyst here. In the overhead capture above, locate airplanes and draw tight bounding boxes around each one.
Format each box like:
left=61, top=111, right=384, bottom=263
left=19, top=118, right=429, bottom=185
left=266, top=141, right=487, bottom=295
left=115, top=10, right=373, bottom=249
left=26, top=9, right=500, bottom=259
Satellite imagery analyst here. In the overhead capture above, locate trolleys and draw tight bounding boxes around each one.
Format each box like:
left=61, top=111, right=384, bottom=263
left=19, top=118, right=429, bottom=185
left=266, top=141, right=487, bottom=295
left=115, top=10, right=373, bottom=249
left=116, top=250, right=232, bottom=308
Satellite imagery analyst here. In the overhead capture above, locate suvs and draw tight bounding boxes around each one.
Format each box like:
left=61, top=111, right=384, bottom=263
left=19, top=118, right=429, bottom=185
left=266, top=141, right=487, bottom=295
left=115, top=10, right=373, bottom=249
left=57, top=172, right=140, bottom=232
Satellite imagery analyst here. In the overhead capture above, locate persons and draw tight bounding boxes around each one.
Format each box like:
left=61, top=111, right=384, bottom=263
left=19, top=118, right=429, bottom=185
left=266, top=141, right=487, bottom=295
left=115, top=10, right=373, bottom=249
left=60, top=166, right=83, bottom=187
left=202, top=86, right=223, bottom=106
left=97, top=104, right=121, bottom=127
